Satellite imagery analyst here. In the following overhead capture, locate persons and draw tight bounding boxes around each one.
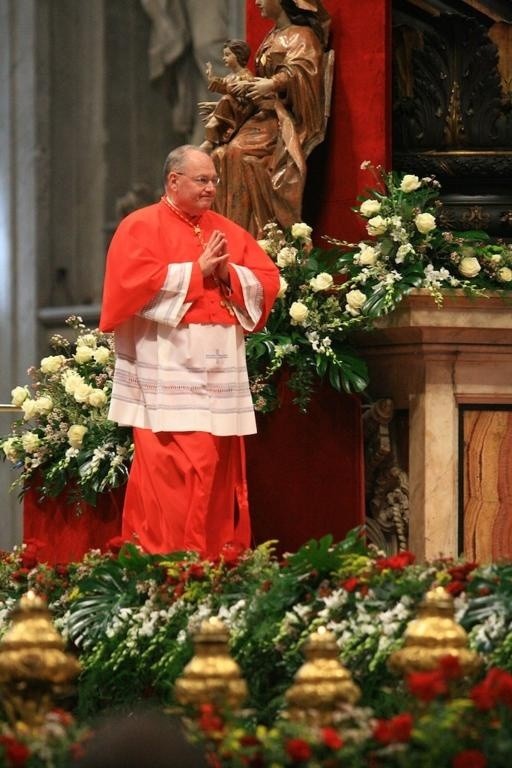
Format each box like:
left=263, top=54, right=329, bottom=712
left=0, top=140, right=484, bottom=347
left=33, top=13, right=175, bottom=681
left=209, top=0, right=326, bottom=249
left=199, top=39, right=260, bottom=153
left=99, top=146, right=280, bottom=572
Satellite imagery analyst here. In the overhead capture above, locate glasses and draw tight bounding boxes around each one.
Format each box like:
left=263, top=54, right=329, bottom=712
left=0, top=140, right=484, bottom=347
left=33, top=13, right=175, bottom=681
left=175, top=171, right=221, bottom=187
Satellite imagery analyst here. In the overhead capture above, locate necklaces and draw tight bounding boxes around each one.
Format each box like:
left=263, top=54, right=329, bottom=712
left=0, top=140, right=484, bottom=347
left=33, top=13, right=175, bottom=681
left=159, top=195, right=219, bottom=287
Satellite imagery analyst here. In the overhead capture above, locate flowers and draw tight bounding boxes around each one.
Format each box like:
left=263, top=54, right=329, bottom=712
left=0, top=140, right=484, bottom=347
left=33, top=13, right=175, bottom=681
left=0, top=525, right=512, bottom=767
left=247, top=162, right=512, bottom=413
left=1, top=316, right=132, bottom=498
